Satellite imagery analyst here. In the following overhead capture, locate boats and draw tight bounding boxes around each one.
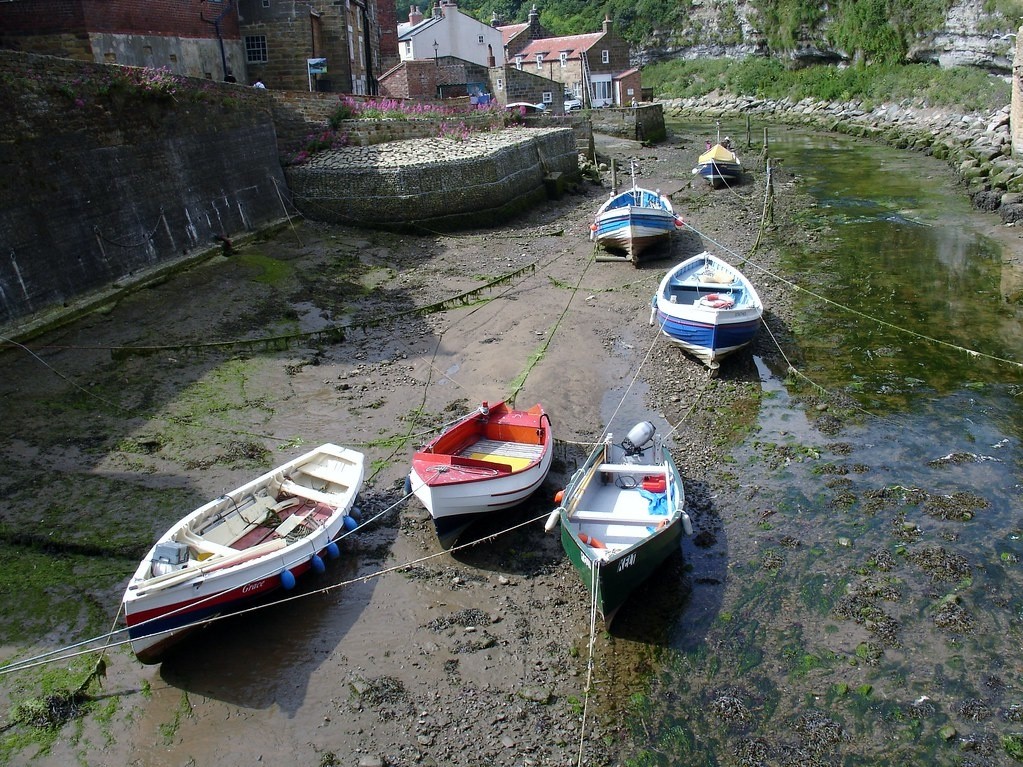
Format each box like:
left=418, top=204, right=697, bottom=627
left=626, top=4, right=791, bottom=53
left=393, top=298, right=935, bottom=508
left=585, top=159, right=678, bottom=266
left=124, top=443, right=365, bottom=662
left=406, top=397, right=553, bottom=524
left=545, top=418, right=690, bottom=632
left=697, top=121, right=745, bottom=189
left=649, top=250, right=763, bottom=376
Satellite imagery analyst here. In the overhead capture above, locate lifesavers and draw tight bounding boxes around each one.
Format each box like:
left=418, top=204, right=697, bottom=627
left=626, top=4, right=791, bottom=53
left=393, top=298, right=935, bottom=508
left=699, top=293, right=735, bottom=309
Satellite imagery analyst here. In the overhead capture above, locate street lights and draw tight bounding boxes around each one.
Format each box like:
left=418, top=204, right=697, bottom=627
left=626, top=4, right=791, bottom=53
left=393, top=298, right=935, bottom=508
left=432, top=40, right=439, bottom=66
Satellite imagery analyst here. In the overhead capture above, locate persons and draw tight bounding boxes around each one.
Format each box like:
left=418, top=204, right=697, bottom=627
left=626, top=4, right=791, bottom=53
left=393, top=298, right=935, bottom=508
left=223, top=70, right=237, bottom=84
left=631, top=96, right=639, bottom=109
left=252, top=77, right=266, bottom=89
left=705, top=138, right=712, bottom=151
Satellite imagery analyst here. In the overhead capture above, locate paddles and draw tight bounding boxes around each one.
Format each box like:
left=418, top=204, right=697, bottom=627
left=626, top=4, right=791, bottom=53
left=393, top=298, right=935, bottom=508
left=127, top=536, right=288, bottom=597
left=565, top=448, right=608, bottom=518
